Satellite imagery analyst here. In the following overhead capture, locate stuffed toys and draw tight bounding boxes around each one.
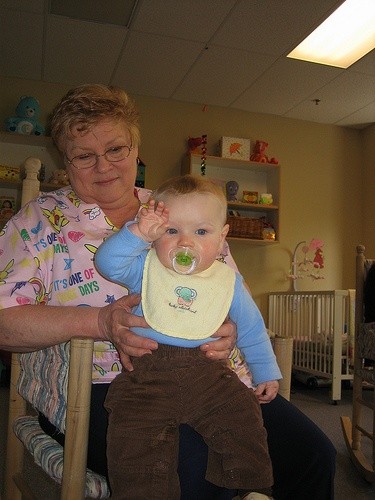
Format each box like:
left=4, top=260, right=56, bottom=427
left=7, top=95, right=45, bottom=136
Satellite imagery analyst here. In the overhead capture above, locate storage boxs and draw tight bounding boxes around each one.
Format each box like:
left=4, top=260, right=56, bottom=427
left=216, top=135, right=251, bottom=162
left=243, top=191, right=258, bottom=204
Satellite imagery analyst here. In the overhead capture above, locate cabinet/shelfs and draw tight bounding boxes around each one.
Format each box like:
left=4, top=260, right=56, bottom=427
left=0, top=130, right=282, bottom=244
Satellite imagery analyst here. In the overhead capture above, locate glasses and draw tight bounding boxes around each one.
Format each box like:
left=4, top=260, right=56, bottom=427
left=64, top=127, right=134, bottom=170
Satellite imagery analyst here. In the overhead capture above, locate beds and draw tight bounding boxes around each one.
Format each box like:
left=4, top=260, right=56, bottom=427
left=267, top=289, right=356, bottom=406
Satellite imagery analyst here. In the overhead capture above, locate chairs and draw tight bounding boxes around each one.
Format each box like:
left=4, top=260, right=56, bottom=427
left=340, top=245, right=375, bottom=483
left=4, top=157, right=294, bottom=500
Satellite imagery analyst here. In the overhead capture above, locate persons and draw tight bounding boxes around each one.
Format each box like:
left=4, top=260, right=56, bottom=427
left=1, top=86, right=336, bottom=500
